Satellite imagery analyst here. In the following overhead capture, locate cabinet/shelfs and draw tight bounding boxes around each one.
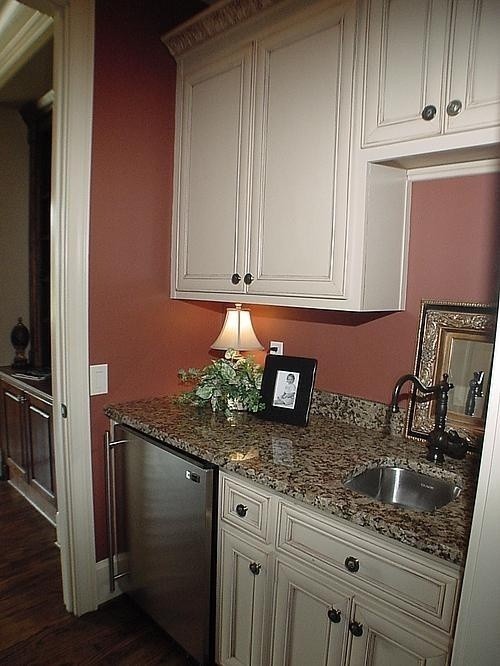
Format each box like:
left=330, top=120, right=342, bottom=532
left=269, top=492, right=463, bottom=666
left=360, top=0, right=499, bottom=181
left=216, top=468, right=272, bottom=666
left=161, top=0, right=412, bottom=313
left=2, top=378, right=57, bottom=509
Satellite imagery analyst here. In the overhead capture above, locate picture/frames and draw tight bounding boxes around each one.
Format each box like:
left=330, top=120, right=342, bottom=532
left=257, top=354, right=318, bottom=427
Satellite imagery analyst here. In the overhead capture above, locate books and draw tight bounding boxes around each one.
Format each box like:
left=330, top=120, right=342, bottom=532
left=10, top=365, right=51, bottom=382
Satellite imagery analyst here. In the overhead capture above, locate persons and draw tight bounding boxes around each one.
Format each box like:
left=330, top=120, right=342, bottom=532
left=276, top=371, right=295, bottom=408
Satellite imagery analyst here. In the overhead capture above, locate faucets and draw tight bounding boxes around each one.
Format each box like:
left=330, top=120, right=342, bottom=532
left=464, top=370, right=484, bottom=416
left=389, top=372, right=469, bottom=461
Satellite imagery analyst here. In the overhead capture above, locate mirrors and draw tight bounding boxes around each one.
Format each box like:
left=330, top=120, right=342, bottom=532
left=404, top=296, right=497, bottom=453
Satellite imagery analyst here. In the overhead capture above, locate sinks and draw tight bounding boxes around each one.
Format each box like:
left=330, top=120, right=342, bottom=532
left=341, top=452, right=462, bottom=513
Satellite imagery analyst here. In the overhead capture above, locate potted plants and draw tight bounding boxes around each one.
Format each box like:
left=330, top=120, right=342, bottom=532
left=168, top=348, right=265, bottom=427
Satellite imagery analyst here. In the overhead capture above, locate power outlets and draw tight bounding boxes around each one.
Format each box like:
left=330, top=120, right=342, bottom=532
left=270, top=340, right=284, bottom=356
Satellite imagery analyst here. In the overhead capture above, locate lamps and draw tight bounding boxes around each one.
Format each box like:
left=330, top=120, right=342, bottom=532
left=210, top=303, right=265, bottom=362
